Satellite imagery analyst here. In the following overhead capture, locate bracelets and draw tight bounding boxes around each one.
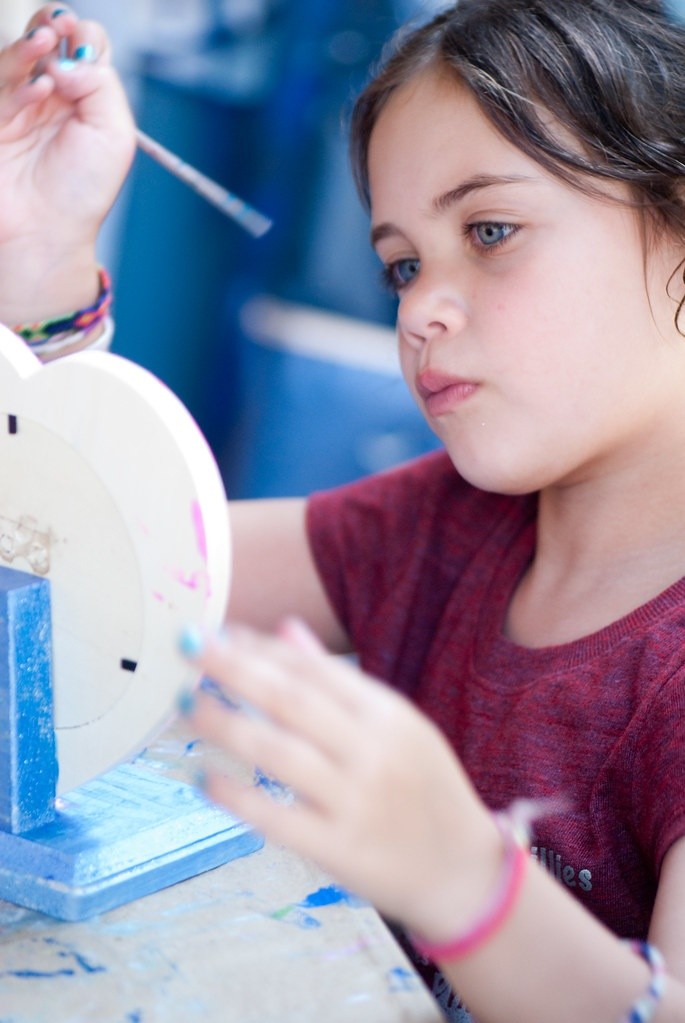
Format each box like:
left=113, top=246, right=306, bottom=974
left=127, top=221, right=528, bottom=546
left=18, top=276, right=112, bottom=352
left=409, top=817, right=524, bottom=965
left=90, top=319, right=117, bottom=352
left=624, top=944, right=666, bottom=1023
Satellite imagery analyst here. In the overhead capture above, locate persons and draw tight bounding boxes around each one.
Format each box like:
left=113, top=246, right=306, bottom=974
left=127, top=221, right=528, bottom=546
left=2, top=0, right=685, bottom=1023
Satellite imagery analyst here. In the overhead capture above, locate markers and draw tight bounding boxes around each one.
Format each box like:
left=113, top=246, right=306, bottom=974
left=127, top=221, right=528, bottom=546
left=130, top=123, right=279, bottom=242
